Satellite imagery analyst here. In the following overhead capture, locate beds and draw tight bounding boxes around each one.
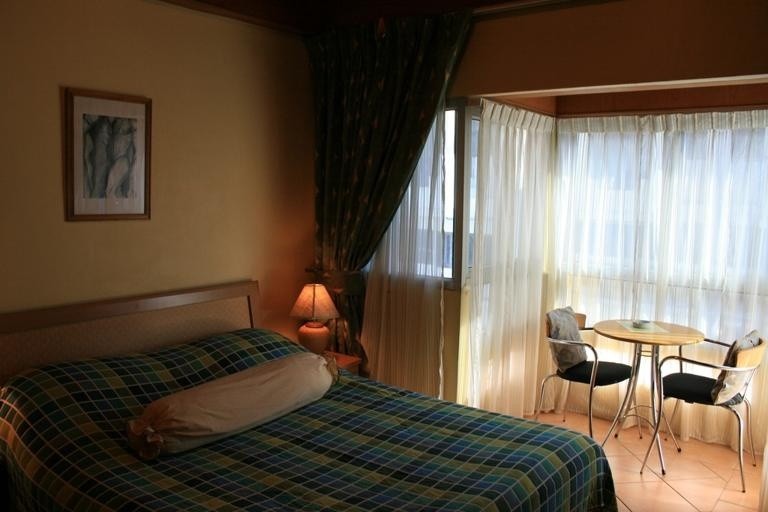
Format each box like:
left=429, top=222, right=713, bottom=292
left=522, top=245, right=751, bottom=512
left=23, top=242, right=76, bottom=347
left=0, top=277, right=609, bottom=512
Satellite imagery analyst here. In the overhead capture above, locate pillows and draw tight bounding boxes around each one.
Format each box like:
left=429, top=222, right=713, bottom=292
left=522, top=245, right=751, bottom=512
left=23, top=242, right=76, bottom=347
left=125, top=350, right=344, bottom=460
left=543, top=305, right=588, bottom=373
left=711, top=329, right=759, bottom=404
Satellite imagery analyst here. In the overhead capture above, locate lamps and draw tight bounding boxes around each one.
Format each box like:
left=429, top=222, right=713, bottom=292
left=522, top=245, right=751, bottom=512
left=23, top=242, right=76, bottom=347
left=288, top=281, right=342, bottom=357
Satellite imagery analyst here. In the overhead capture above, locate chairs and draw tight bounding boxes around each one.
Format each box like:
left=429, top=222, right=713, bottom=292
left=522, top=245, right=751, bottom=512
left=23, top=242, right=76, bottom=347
left=637, top=330, right=767, bottom=492
left=533, top=305, right=644, bottom=443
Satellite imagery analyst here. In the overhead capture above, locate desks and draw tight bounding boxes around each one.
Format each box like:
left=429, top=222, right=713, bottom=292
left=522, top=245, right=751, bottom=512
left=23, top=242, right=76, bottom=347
left=593, top=318, right=707, bottom=476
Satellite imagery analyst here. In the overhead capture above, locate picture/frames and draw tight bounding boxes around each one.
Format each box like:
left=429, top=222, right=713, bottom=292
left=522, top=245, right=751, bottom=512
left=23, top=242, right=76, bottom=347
left=63, top=83, right=153, bottom=223
